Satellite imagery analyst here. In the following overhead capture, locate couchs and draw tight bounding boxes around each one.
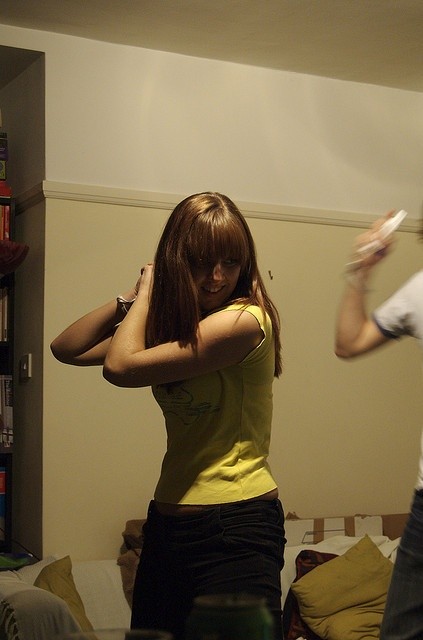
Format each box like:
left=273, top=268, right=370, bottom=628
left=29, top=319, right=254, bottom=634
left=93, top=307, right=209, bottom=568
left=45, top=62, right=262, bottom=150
left=114, top=512, right=423, bottom=639
left=0, top=555, right=173, bottom=639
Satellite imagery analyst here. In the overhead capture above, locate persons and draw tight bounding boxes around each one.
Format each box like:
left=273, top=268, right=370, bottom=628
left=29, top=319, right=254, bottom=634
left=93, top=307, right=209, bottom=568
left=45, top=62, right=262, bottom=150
left=50, top=191, right=286, bottom=640
left=333, top=208, right=421, bottom=636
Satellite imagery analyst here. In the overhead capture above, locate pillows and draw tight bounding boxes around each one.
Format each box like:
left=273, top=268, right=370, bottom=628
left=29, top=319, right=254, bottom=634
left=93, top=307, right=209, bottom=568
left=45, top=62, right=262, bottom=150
left=290, top=532, right=396, bottom=639
left=34, top=556, right=98, bottom=637
left=283, top=550, right=341, bottom=640
